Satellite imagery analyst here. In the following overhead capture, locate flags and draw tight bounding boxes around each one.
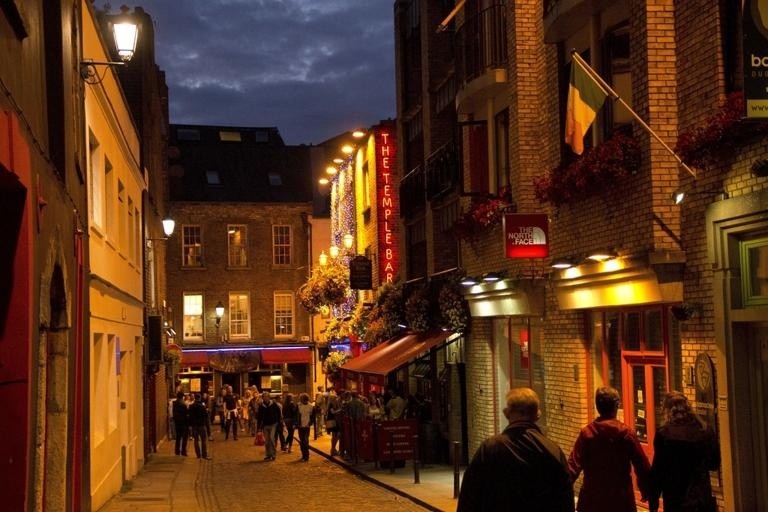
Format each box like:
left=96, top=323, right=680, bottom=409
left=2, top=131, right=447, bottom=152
left=562, top=55, right=610, bottom=156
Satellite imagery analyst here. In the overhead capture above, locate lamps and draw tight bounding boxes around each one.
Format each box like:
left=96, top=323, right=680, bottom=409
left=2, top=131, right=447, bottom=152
left=458, top=274, right=481, bottom=286
left=481, top=270, right=508, bottom=281
left=671, top=189, right=726, bottom=205
left=315, top=125, right=368, bottom=266
left=145, top=217, right=176, bottom=241
left=585, top=248, right=616, bottom=262
left=548, top=256, right=577, bottom=270
left=213, top=300, right=226, bottom=328
left=77, top=6, right=141, bottom=86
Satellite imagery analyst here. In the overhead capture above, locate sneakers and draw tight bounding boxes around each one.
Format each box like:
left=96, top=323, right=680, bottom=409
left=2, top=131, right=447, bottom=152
left=203, top=455, right=213, bottom=461
left=264, top=455, right=277, bottom=461
left=282, top=444, right=295, bottom=453
left=207, top=436, right=214, bottom=440
left=224, top=435, right=238, bottom=441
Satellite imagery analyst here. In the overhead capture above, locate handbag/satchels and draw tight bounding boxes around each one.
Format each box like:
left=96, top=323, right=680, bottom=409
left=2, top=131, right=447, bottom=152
left=254, top=429, right=266, bottom=446
left=326, top=420, right=338, bottom=429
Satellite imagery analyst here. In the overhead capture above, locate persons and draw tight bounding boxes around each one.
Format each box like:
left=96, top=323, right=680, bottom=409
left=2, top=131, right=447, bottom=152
left=173, top=380, right=427, bottom=463
left=649, top=391, right=720, bottom=512
left=456, top=388, right=574, bottom=512
left=566, top=387, right=652, bottom=512
